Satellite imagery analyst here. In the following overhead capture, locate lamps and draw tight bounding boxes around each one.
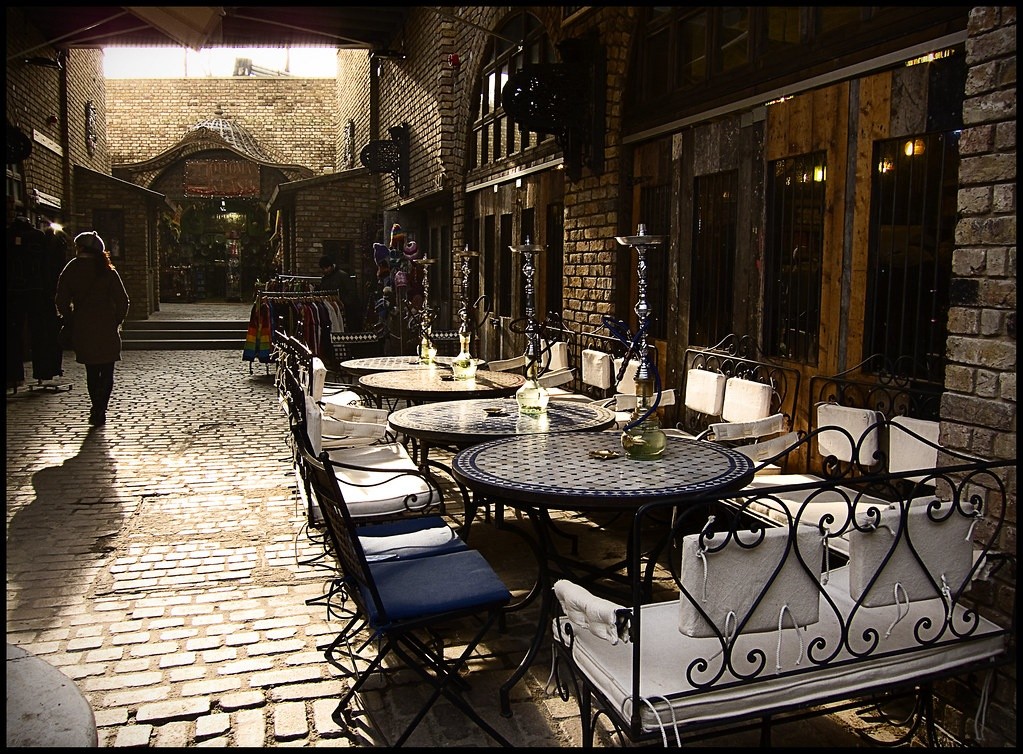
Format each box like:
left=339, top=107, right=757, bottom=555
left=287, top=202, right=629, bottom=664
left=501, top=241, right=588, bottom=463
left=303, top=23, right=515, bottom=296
left=20, top=58, right=63, bottom=71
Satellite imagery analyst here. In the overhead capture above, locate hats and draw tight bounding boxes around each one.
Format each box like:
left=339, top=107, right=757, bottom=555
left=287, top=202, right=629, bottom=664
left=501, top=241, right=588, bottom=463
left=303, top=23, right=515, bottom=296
left=368, top=225, right=424, bottom=323
left=319, top=256, right=334, bottom=267
left=72, top=231, right=104, bottom=252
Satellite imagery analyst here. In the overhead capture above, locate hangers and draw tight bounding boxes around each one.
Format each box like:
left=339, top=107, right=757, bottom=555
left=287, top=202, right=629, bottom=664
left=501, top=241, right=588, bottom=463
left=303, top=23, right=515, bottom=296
left=263, top=290, right=344, bottom=314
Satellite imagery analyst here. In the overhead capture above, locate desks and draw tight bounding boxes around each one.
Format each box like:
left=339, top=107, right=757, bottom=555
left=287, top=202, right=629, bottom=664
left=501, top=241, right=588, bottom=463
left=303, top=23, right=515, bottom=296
left=7, top=644, right=99, bottom=748
left=359, top=369, right=526, bottom=461
left=388, top=399, right=616, bottom=631
left=340, top=356, right=486, bottom=409
left=453, top=433, right=755, bottom=717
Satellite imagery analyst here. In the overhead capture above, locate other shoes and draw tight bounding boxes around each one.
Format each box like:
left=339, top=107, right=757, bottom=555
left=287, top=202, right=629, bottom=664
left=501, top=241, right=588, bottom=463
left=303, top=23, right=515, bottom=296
left=88, top=414, right=105, bottom=426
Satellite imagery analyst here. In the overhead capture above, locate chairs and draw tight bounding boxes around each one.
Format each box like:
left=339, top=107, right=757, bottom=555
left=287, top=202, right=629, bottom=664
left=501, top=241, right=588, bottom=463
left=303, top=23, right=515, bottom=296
left=268, top=330, right=511, bottom=748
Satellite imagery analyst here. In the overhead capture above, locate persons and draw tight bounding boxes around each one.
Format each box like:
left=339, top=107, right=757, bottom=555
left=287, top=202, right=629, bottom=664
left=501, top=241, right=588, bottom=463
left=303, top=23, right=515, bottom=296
left=55, top=231, right=129, bottom=425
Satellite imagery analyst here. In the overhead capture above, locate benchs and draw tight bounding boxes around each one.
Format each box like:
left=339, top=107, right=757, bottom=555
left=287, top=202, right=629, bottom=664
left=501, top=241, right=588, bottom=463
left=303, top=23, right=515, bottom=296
left=487, top=338, right=1006, bottom=748
